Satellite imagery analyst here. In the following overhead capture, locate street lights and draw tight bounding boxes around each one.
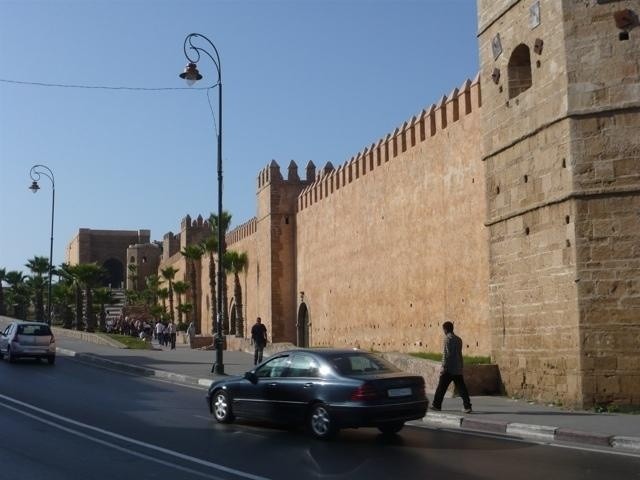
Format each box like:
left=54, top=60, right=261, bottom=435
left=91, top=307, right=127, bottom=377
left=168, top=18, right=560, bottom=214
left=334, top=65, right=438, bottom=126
left=30, top=163, right=56, bottom=326
left=180, top=31, right=226, bottom=374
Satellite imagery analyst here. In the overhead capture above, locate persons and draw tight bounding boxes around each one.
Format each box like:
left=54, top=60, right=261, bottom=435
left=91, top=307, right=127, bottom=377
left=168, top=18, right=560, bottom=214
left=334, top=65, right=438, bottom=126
left=250, top=317, right=267, bottom=365
left=111, top=314, right=196, bottom=351
left=206, top=328, right=218, bottom=349
left=430, top=322, right=472, bottom=413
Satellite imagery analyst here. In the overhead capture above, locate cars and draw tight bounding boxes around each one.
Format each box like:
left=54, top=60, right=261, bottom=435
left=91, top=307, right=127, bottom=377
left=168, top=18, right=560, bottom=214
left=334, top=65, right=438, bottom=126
left=205, top=348, right=430, bottom=443
left=0, top=321, right=56, bottom=364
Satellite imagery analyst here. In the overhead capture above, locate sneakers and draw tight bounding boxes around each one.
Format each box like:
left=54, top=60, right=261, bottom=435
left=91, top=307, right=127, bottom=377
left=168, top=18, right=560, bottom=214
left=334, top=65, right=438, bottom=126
left=428, top=405, right=442, bottom=412
left=462, top=408, right=472, bottom=413
